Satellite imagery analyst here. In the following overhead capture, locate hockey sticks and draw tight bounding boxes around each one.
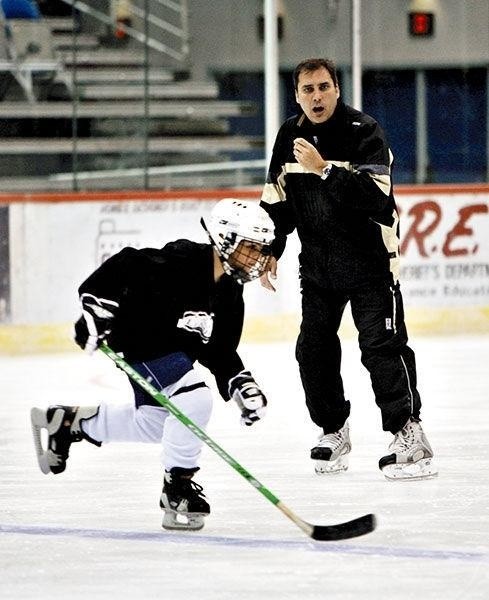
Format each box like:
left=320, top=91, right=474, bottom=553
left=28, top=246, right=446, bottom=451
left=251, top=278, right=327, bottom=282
left=97, top=341, right=376, bottom=541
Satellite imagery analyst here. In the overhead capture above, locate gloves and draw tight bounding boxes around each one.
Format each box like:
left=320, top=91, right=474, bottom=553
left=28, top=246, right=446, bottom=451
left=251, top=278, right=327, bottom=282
left=75, top=293, right=120, bottom=352
left=229, top=369, right=267, bottom=428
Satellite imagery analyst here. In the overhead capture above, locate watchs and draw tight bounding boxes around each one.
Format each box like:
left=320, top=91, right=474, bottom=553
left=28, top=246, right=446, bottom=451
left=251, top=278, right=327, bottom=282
left=318, top=162, right=333, bottom=182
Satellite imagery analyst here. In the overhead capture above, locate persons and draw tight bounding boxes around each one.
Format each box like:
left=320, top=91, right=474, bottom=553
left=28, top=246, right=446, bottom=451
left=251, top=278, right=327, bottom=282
left=42, top=196, right=278, bottom=520
left=256, top=58, right=436, bottom=472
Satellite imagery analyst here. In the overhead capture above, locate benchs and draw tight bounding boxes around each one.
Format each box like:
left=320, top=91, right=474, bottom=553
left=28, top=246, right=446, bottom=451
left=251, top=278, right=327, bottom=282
left=0, top=1, right=270, bottom=194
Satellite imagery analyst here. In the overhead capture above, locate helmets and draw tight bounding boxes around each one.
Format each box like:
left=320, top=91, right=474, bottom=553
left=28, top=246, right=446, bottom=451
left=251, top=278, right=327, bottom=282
left=200, top=197, right=275, bottom=286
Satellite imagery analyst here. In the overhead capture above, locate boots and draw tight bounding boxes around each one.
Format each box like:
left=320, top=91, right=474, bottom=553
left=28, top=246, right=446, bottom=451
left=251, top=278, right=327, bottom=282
left=311, top=421, right=352, bottom=461
left=377, top=420, right=434, bottom=469
left=160, top=466, right=211, bottom=515
left=46, top=403, right=101, bottom=475
